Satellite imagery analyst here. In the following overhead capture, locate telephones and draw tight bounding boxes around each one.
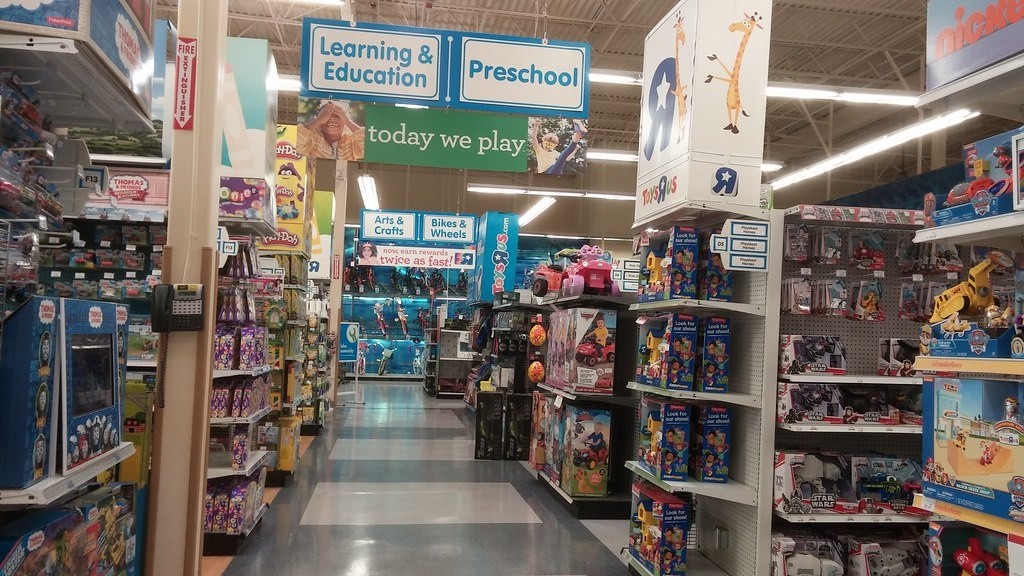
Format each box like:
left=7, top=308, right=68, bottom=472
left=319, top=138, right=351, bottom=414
left=151, top=283, right=205, bottom=333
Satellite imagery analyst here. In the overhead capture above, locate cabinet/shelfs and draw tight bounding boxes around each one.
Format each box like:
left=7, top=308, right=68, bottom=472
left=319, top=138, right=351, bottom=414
left=0, top=0, right=278, bottom=576
left=421, top=296, right=486, bottom=400
left=342, top=228, right=468, bottom=378
left=463, top=301, right=545, bottom=413
left=622, top=203, right=925, bottom=576
left=909, top=52, right=1024, bottom=576
left=530, top=292, right=633, bottom=520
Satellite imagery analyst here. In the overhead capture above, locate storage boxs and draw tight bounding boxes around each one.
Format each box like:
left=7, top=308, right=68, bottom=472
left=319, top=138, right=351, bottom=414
left=769, top=449, right=928, bottom=576
left=635, top=225, right=733, bottom=303
left=218, top=176, right=279, bottom=231
left=920, top=377, right=1024, bottom=523
left=529, top=390, right=612, bottom=498
left=962, top=125, right=1024, bottom=212
left=777, top=382, right=922, bottom=426
left=628, top=482, right=688, bottom=576
left=0, top=0, right=154, bottom=120
left=474, top=391, right=533, bottom=462
left=545, top=307, right=618, bottom=396
left=254, top=255, right=314, bottom=471
left=931, top=193, right=1013, bottom=227
left=637, top=396, right=732, bottom=484
left=636, top=312, right=731, bottom=393
left=0, top=482, right=137, bottom=576
left=927, top=520, right=1024, bottom=576
left=779, top=334, right=848, bottom=375
left=929, top=324, right=1014, bottom=358
left=0, top=293, right=131, bottom=490
left=25, top=480, right=105, bottom=511
left=276, top=254, right=281, bottom=268
left=876, top=338, right=926, bottom=377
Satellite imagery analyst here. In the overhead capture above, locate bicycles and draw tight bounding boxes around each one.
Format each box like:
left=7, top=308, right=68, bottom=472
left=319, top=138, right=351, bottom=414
left=415, top=307, right=429, bottom=326
left=412, top=346, right=428, bottom=375
left=394, top=300, right=408, bottom=336
left=377, top=342, right=397, bottom=375
left=344, top=259, right=468, bottom=295
left=370, top=301, right=389, bottom=338
left=356, top=341, right=376, bottom=375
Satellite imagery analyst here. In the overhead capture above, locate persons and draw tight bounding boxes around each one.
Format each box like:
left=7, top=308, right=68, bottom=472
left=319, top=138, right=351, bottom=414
left=585, top=317, right=608, bottom=358
left=296, top=102, right=364, bottom=161
left=532, top=118, right=588, bottom=174
left=580, top=422, right=605, bottom=455
left=358, top=242, right=379, bottom=265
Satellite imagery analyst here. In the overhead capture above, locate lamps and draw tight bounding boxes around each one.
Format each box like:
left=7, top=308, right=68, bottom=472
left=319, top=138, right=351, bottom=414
left=588, top=67, right=920, bottom=107
left=466, top=182, right=637, bottom=202
left=517, top=195, right=557, bottom=228
left=767, top=107, right=981, bottom=192
left=357, top=173, right=380, bottom=212
left=586, top=147, right=784, bottom=173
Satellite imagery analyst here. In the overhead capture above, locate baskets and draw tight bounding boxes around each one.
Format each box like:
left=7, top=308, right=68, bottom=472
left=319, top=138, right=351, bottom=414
left=383, top=348, right=393, bottom=358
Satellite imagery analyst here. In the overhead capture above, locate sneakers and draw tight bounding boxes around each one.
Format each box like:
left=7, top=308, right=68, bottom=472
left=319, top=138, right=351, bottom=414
left=573, top=118, right=588, bottom=138
